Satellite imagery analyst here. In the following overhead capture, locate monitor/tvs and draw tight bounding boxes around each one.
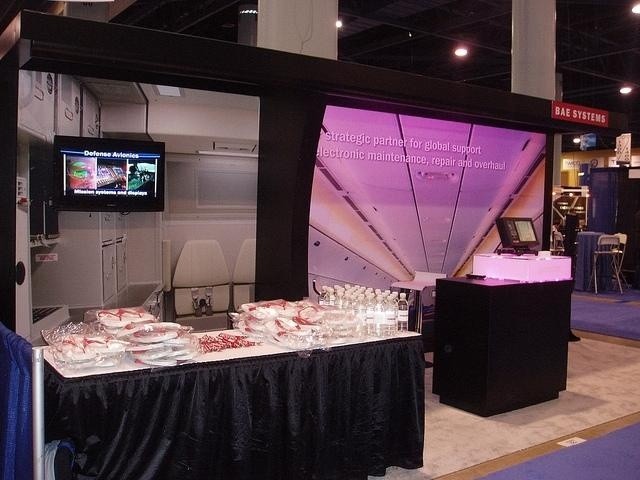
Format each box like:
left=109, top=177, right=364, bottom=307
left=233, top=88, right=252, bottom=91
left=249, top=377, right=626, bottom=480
left=52, top=135, right=165, bottom=212
left=579, top=132, right=602, bottom=151
left=495, top=217, right=541, bottom=255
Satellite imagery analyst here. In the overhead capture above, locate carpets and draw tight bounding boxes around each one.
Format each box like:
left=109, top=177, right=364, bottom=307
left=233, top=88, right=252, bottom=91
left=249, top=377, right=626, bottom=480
left=376, top=338, right=640, bottom=480
left=573, top=290, right=640, bottom=340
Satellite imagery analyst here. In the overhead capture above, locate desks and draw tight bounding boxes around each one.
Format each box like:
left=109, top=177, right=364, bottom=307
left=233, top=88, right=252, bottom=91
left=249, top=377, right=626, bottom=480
left=431, top=271, right=572, bottom=419
left=575, top=231, right=608, bottom=292
left=28, top=308, right=423, bottom=477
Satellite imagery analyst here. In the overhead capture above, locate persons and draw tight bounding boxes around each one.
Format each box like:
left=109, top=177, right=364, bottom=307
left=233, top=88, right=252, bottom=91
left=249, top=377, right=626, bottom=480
left=553, top=219, right=560, bottom=232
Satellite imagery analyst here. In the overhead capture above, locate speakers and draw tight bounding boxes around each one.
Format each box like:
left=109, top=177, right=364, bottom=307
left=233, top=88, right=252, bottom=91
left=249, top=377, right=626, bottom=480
left=580, top=163, right=592, bottom=186
left=30, top=200, right=59, bottom=240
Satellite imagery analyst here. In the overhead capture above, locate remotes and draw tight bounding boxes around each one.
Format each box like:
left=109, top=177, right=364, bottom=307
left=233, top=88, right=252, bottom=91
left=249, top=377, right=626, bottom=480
left=466, top=273, right=486, bottom=278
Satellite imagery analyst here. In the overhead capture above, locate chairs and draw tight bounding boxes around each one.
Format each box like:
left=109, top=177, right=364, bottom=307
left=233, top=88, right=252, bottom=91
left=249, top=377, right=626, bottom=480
left=586, top=232, right=630, bottom=296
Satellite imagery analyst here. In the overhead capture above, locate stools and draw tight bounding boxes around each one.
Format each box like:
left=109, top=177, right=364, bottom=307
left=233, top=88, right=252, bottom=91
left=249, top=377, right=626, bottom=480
left=391, top=268, right=445, bottom=367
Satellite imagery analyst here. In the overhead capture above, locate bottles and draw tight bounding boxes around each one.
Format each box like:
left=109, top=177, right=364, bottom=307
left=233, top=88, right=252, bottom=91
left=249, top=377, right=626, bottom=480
left=317, top=284, right=409, bottom=340
